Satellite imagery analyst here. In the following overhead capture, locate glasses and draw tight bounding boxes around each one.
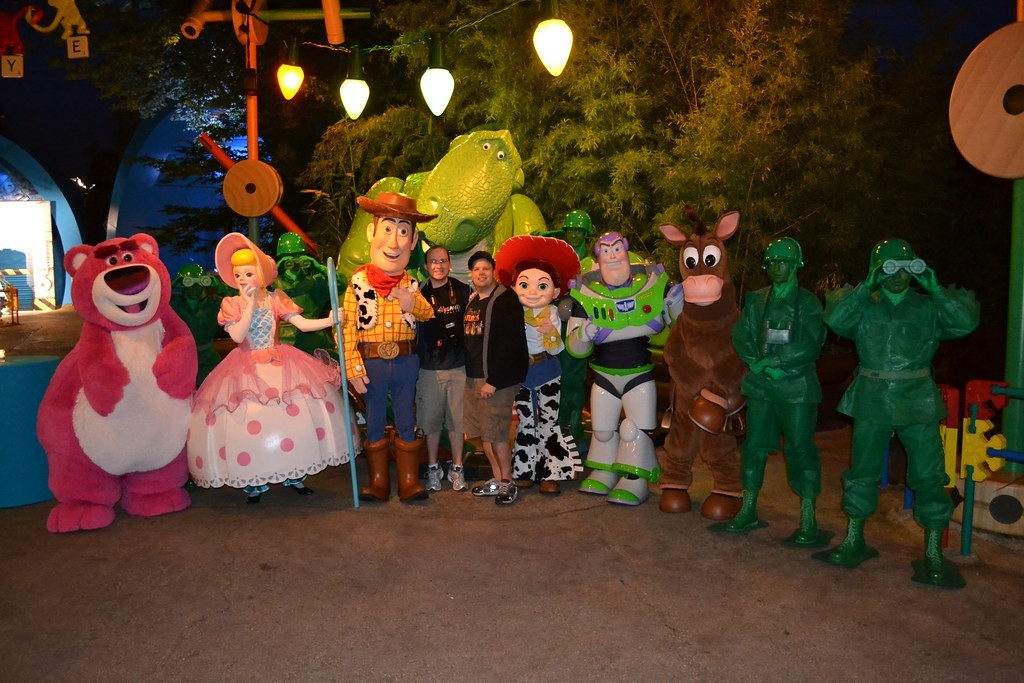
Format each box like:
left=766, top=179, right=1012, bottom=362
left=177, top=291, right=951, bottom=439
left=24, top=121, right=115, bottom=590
left=427, top=259, right=448, bottom=264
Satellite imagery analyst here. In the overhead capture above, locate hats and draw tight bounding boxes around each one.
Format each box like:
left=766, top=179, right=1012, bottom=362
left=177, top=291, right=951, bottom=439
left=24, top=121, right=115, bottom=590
left=467, top=251, right=495, bottom=271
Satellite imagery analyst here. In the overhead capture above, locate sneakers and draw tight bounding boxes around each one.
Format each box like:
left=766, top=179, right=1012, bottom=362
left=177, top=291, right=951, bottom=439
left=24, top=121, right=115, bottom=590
left=494, top=479, right=518, bottom=504
left=446, top=464, right=469, bottom=491
left=471, top=478, right=502, bottom=496
left=424, top=462, right=444, bottom=492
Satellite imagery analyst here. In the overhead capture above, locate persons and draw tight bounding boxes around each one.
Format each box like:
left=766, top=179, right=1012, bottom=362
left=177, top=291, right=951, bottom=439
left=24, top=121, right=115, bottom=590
left=415, top=246, right=473, bottom=493
left=724, top=236, right=827, bottom=546
left=823, top=237, right=983, bottom=579
left=494, top=235, right=581, bottom=495
left=185, top=231, right=363, bottom=504
left=333, top=190, right=441, bottom=503
left=460, top=252, right=530, bottom=505
left=565, top=231, right=679, bottom=501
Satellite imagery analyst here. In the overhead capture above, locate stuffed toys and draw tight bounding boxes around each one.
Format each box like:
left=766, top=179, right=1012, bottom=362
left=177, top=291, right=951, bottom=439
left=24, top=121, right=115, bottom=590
left=657, top=208, right=753, bottom=521
left=37, top=233, right=200, bottom=534
